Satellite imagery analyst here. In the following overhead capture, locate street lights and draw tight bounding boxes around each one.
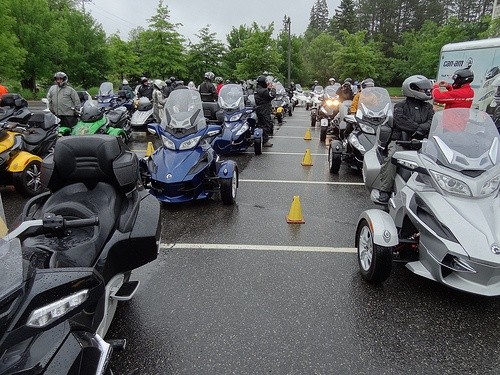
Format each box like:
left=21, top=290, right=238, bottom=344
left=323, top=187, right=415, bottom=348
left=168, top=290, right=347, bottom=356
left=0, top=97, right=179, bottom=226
left=283, top=17, right=291, bottom=88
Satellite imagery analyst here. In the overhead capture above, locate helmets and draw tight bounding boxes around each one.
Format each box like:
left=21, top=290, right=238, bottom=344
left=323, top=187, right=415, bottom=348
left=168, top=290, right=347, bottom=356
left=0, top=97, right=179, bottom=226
left=328, top=78, right=335, bottom=85
left=204, top=71, right=215, bottom=82
left=257, top=75, right=270, bottom=88
left=344, top=78, right=352, bottom=83
left=226, top=79, right=231, bottom=84
left=401, top=75, right=433, bottom=101
left=53, top=71, right=69, bottom=87
left=290, top=82, right=295, bottom=87
left=169, top=76, right=176, bottom=81
left=342, top=82, right=351, bottom=93
left=140, top=77, right=148, bottom=84
left=452, top=68, right=474, bottom=89
left=165, top=79, right=172, bottom=84
left=357, top=82, right=361, bottom=91
left=215, top=76, right=223, bottom=84
left=491, top=75, right=500, bottom=105
left=122, top=79, right=129, bottom=85
left=361, top=78, right=375, bottom=89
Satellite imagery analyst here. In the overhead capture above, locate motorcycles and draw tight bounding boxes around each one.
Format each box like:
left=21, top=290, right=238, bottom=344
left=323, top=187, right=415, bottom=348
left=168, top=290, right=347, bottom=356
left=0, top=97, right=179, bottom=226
left=0, top=111, right=61, bottom=197
left=210, top=84, right=263, bottom=155
left=0, top=134, right=162, bottom=375
left=320, top=100, right=353, bottom=141
left=471, top=66, right=500, bottom=133
left=328, top=87, right=394, bottom=173
left=42, top=92, right=92, bottom=126
left=93, top=80, right=200, bottom=137
left=175, top=78, right=305, bottom=136
left=0, top=93, right=31, bottom=124
left=58, top=99, right=134, bottom=145
left=139, top=89, right=239, bottom=205
left=355, top=108, right=500, bottom=296
left=311, top=90, right=340, bottom=127
left=305, top=86, right=323, bottom=110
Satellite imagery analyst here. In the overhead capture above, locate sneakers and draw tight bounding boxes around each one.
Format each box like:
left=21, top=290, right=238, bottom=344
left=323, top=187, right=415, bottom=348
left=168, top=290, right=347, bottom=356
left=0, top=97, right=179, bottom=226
left=263, top=142, right=273, bottom=147
left=379, top=191, right=389, bottom=202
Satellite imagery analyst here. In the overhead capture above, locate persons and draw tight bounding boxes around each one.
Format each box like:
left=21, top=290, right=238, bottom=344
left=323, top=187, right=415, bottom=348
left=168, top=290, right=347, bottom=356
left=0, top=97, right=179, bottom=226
left=215, top=77, right=225, bottom=95
left=312, top=80, right=319, bottom=92
left=330, top=78, right=362, bottom=119
left=343, top=78, right=376, bottom=139
left=287, top=82, right=296, bottom=103
left=162, top=77, right=189, bottom=98
left=254, top=75, right=276, bottom=147
left=119, top=80, right=132, bottom=90
left=432, top=69, right=474, bottom=132
left=47, top=72, right=81, bottom=129
left=199, top=71, right=218, bottom=102
left=225, top=79, right=256, bottom=98
left=378, top=75, right=435, bottom=202
left=152, top=79, right=169, bottom=123
left=137, top=77, right=154, bottom=101
left=329, top=78, right=336, bottom=85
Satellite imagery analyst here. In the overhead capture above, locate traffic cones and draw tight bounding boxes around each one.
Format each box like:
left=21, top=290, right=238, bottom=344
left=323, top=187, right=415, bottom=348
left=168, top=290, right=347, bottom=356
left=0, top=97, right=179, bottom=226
left=286, top=195, right=306, bottom=224
left=304, top=127, right=312, bottom=140
left=145, top=142, right=154, bottom=157
left=301, top=148, right=313, bottom=166
